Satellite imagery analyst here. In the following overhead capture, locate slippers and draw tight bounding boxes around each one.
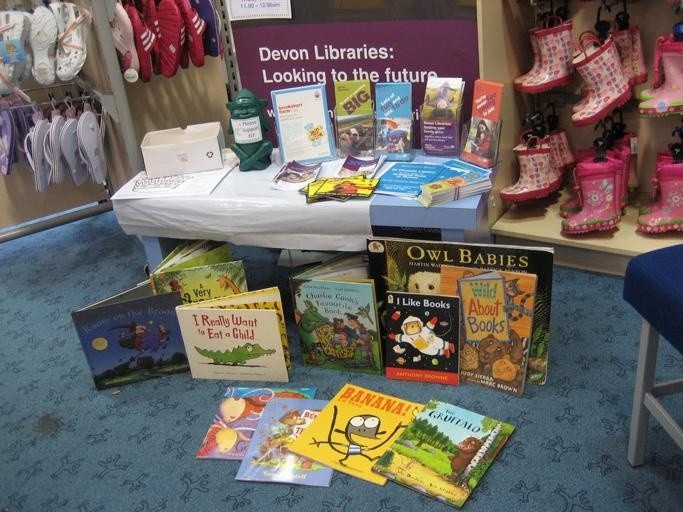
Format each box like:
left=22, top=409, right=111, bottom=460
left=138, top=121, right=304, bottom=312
left=0, top=0, right=109, bottom=194
left=112, top=1, right=221, bottom=84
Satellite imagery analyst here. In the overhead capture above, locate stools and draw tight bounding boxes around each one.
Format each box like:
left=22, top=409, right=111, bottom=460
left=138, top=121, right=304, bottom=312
left=622, top=243, right=681, bottom=466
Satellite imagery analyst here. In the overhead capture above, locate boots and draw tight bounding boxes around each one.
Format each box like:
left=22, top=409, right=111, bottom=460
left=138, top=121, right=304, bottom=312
left=500, top=128, right=682, bottom=236
left=514, top=16, right=683, bottom=124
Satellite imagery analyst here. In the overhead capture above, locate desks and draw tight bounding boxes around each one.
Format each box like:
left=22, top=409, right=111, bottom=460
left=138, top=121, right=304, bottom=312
left=112, top=146, right=498, bottom=274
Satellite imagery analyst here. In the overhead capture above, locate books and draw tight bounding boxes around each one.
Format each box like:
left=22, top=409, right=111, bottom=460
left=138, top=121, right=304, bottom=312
left=198, top=384, right=516, bottom=508
left=70, top=235, right=553, bottom=396
left=269, top=83, right=334, bottom=162
